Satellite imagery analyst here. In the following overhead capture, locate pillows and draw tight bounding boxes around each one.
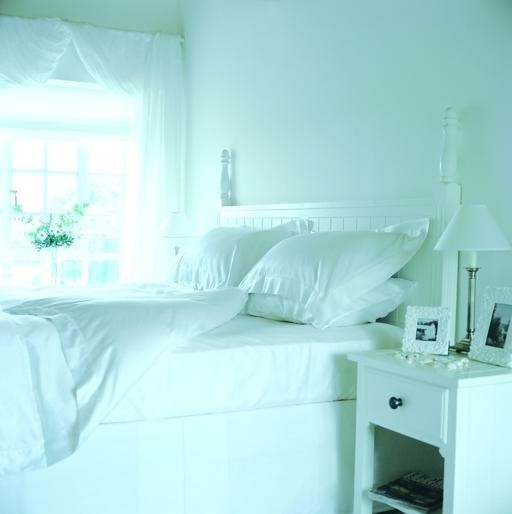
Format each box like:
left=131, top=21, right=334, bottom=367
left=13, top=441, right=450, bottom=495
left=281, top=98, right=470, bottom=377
left=167, top=215, right=430, bottom=333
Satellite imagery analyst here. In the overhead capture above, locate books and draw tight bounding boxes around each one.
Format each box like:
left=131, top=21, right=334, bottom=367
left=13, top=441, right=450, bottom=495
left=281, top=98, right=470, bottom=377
left=368, top=468, right=443, bottom=514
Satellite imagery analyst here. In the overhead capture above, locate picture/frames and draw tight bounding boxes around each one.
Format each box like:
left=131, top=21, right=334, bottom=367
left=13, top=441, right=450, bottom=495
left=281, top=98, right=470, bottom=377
left=468, top=286, right=512, bottom=368
left=401, top=305, right=451, bottom=355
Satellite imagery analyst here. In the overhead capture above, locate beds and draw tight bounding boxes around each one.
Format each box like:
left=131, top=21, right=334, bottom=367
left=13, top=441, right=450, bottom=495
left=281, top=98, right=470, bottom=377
left=0, top=104, right=462, bottom=514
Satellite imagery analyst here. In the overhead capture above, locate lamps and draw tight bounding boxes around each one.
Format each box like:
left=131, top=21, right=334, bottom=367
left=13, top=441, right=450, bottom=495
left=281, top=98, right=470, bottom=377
left=433, top=202, right=512, bottom=353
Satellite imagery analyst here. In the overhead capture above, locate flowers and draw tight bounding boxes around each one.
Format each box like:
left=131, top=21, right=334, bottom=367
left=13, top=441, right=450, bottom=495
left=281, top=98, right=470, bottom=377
left=12, top=194, right=91, bottom=251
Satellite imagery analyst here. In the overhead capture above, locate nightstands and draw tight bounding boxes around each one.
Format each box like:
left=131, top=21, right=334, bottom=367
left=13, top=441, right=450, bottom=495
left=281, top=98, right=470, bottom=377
left=345, top=349, right=512, bottom=513
